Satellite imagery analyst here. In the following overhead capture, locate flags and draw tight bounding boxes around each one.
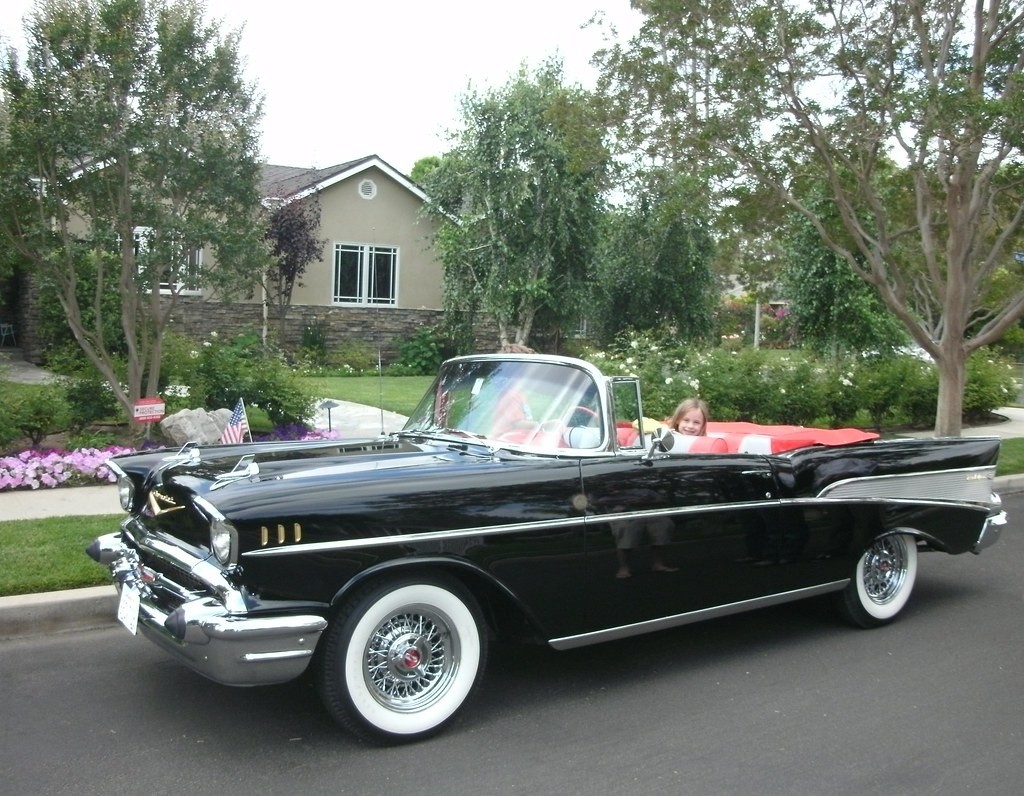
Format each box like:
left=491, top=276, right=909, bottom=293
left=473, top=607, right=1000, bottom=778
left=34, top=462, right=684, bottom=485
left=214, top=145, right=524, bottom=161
left=220, top=398, right=248, bottom=445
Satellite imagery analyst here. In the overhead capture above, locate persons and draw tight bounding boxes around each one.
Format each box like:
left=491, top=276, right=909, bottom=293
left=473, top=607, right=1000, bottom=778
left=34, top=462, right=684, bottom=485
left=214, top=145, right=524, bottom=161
left=605, top=500, right=683, bottom=580
left=632, top=397, right=708, bottom=437
left=490, top=343, right=541, bottom=436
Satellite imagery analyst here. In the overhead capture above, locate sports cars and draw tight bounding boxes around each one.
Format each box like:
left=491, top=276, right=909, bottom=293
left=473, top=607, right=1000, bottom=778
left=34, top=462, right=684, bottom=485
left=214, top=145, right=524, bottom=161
left=82, top=352, right=1008, bottom=749
